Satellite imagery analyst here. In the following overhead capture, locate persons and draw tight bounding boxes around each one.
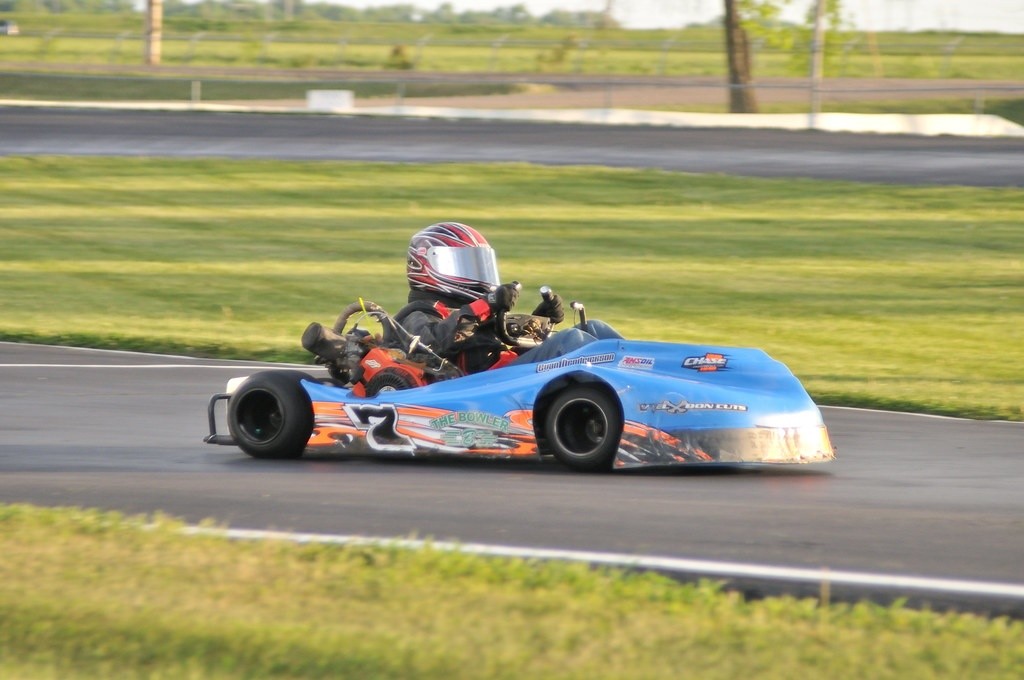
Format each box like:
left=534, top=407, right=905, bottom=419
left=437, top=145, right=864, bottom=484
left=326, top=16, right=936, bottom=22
left=392, top=221, right=623, bottom=375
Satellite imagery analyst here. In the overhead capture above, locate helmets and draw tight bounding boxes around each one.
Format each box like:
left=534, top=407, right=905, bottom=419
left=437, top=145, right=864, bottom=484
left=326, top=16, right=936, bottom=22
left=407, top=223, right=497, bottom=301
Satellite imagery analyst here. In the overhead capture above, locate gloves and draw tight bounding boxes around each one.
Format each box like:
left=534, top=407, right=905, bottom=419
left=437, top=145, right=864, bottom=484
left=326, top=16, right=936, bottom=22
left=532, top=295, right=565, bottom=323
left=484, top=283, right=519, bottom=312
